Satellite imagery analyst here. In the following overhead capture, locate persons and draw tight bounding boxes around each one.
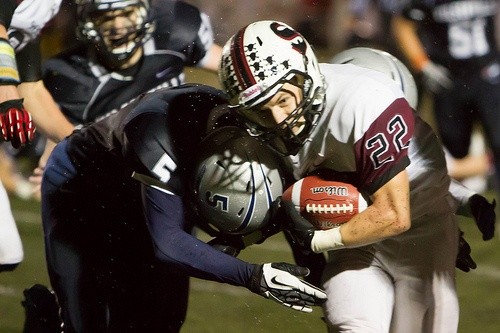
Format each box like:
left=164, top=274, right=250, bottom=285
left=22, top=83, right=329, bottom=333
left=217, top=19, right=459, bottom=333
left=0, top=0, right=500, bottom=272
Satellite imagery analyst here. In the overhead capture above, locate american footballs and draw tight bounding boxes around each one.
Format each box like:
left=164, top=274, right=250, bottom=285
left=283, top=176, right=369, bottom=231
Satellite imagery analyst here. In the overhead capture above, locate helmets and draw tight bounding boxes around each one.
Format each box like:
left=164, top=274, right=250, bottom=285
left=188, top=134, right=285, bottom=237
left=74, top=0, right=157, bottom=72
left=332, top=47, right=418, bottom=113
left=216, top=19, right=329, bottom=158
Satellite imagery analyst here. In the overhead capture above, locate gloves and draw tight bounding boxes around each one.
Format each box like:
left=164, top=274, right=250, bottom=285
left=415, top=60, right=457, bottom=97
left=469, top=194, right=496, bottom=241
left=0, top=98, right=37, bottom=148
left=268, top=196, right=320, bottom=255
left=456, top=227, right=477, bottom=272
left=247, top=262, right=328, bottom=313
